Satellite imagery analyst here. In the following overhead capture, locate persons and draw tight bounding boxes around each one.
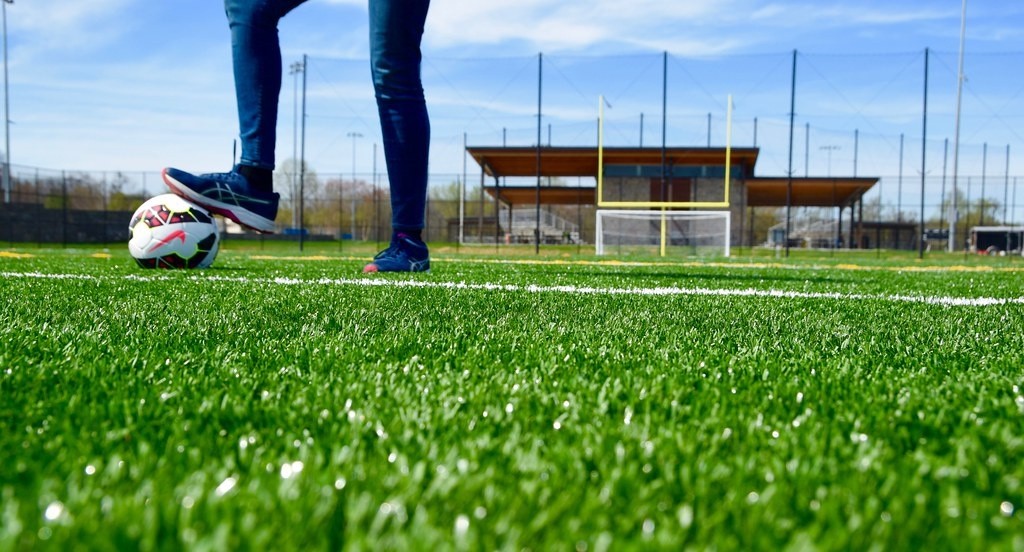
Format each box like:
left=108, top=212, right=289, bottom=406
left=162, top=0, right=430, bottom=272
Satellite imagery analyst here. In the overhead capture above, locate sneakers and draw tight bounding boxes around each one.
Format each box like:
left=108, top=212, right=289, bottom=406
left=362, top=235, right=431, bottom=275
left=162, top=167, right=280, bottom=235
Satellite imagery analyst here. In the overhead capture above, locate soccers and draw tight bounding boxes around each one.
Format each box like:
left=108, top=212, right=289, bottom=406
left=127, top=192, right=220, bottom=269
